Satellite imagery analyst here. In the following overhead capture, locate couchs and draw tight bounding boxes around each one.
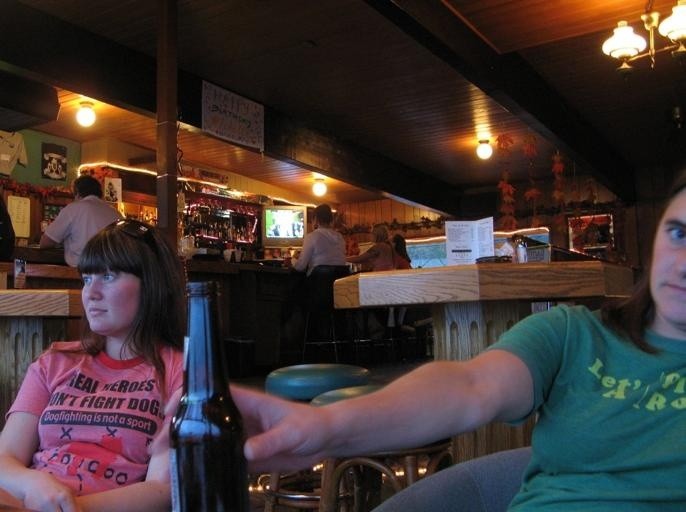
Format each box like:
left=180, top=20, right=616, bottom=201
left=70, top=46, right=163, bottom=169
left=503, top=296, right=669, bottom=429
left=371, top=445, right=533, bottom=511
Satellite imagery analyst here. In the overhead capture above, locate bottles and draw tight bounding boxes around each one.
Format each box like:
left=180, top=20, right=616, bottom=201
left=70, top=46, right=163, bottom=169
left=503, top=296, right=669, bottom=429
left=169, top=275, right=250, bottom=512
left=184, top=206, right=249, bottom=242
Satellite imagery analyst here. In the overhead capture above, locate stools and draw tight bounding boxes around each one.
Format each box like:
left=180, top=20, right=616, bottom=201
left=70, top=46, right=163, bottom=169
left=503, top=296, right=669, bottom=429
left=308, top=385, right=452, bottom=511
left=258, top=363, right=371, bottom=511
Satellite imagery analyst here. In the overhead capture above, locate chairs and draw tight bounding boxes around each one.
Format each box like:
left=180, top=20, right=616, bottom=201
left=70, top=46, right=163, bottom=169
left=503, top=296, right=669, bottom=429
left=280, top=263, right=351, bottom=363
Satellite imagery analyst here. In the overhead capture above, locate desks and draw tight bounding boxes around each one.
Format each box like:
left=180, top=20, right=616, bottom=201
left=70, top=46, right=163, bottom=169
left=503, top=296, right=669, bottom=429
left=332, top=260, right=636, bottom=467
left=1, top=287, right=85, bottom=435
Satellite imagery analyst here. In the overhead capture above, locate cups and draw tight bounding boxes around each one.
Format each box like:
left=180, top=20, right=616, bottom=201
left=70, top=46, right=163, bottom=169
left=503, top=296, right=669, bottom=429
left=290, top=249, right=300, bottom=260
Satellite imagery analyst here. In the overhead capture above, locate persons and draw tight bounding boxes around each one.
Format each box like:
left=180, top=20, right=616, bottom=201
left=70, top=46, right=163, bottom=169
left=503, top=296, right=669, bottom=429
left=286, top=201, right=345, bottom=276
left=0, top=216, right=186, bottom=512
left=39, top=175, right=126, bottom=269
left=0, top=196, right=15, bottom=260
left=386, top=232, right=416, bottom=342
left=345, top=220, right=394, bottom=342
left=144, top=174, right=686, bottom=512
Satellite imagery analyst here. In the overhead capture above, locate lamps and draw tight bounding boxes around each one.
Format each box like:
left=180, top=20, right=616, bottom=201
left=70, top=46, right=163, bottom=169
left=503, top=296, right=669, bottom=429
left=473, top=136, right=494, bottom=161
left=312, top=177, right=328, bottom=197
left=75, top=101, right=96, bottom=126
left=602, top=1, right=686, bottom=91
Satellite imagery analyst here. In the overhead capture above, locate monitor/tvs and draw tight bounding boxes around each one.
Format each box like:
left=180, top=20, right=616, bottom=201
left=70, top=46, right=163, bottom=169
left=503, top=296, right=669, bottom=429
left=261, top=206, right=307, bottom=248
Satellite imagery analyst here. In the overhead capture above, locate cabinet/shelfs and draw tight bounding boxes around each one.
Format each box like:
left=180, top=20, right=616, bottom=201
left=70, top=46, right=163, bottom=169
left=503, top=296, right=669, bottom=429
left=0, top=164, right=265, bottom=263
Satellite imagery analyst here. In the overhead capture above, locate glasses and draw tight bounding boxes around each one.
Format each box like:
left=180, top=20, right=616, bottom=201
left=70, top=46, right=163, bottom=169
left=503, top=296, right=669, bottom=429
left=108, top=218, right=159, bottom=257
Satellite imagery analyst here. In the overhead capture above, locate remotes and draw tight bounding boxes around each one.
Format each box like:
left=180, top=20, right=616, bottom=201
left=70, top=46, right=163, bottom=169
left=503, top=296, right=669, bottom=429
left=475, top=255, right=513, bottom=263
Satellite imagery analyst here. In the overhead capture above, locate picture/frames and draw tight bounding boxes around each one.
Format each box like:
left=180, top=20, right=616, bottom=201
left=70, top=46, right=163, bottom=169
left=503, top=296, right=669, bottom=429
left=564, top=210, right=618, bottom=255
